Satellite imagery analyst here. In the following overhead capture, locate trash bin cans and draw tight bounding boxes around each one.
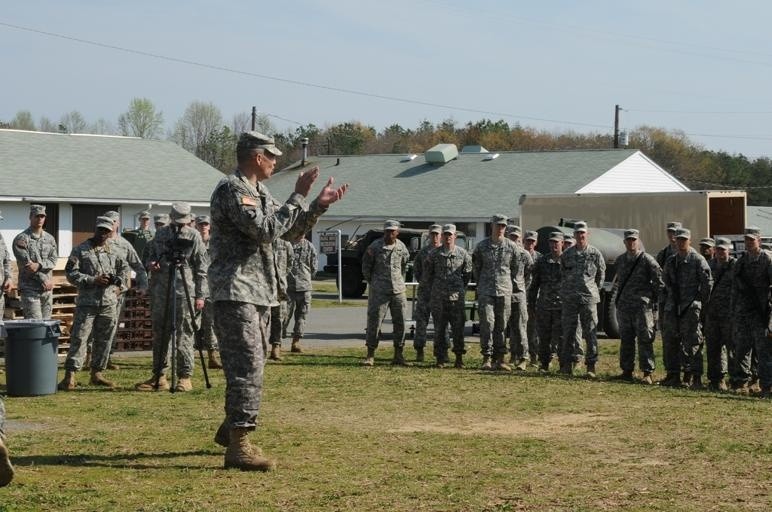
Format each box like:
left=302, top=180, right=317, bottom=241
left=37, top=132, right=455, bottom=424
left=3, top=319, right=63, bottom=395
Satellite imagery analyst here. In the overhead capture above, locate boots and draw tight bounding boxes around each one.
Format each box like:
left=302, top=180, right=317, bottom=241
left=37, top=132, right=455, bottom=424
left=614, top=367, right=771, bottom=398
left=175, top=375, right=193, bottom=392
left=79, top=358, right=120, bottom=371
left=206, top=337, right=304, bottom=369
left=134, top=373, right=170, bottom=392
left=87, top=365, right=115, bottom=388
left=213, top=416, right=276, bottom=470
left=57, top=370, right=75, bottom=390
left=358, top=344, right=605, bottom=381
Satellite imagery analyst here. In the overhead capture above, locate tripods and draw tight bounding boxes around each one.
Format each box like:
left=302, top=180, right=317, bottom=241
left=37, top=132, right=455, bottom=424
left=154, top=265, right=211, bottom=393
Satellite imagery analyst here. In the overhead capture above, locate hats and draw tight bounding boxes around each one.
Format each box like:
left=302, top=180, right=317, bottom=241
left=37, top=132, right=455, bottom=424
left=573, top=221, right=588, bottom=233
left=236, top=129, right=283, bottom=156
left=547, top=230, right=574, bottom=245
left=168, top=201, right=193, bottom=225
left=30, top=204, right=47, bottom=217
left=382, top=219, right=401, bottom=231
left=623, top=229, right=640, bottom=239
left=104, top=210, right=210, bottom=227
left=699, top=238, right=734, bottom=250
left=492, top=213, right=509, bottom=226
left=95, top=215, right=115, bottom=232
left=664, top=222, right=691, bottom=240
left=506, top=222, right=539, bottom=241
left=428, top=223, right=457, bottom=235
left=742, top=226, right=761, bottom=240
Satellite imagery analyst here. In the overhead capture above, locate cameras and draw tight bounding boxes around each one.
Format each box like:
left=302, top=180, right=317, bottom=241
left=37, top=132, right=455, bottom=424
left=107, top=274, right=122, bottom=287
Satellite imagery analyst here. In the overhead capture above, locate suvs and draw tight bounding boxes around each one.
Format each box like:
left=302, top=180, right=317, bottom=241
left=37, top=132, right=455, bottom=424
left=323, top=224, right=473, bottom=302
